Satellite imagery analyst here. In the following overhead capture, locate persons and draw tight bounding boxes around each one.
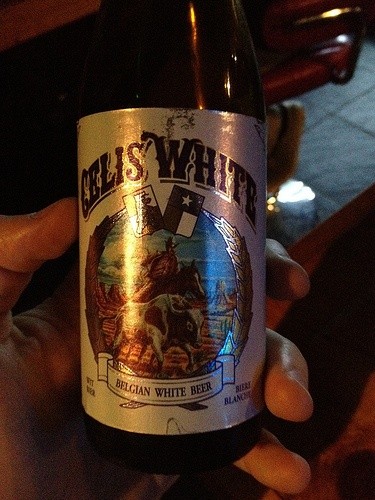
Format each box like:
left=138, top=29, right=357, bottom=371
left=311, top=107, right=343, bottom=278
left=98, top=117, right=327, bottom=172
left=1, top=196, right=316, bottom=500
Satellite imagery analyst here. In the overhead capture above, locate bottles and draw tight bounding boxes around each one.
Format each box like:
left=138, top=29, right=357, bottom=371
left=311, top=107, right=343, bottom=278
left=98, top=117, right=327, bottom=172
left=73, top=1, right=270, bottom=477
